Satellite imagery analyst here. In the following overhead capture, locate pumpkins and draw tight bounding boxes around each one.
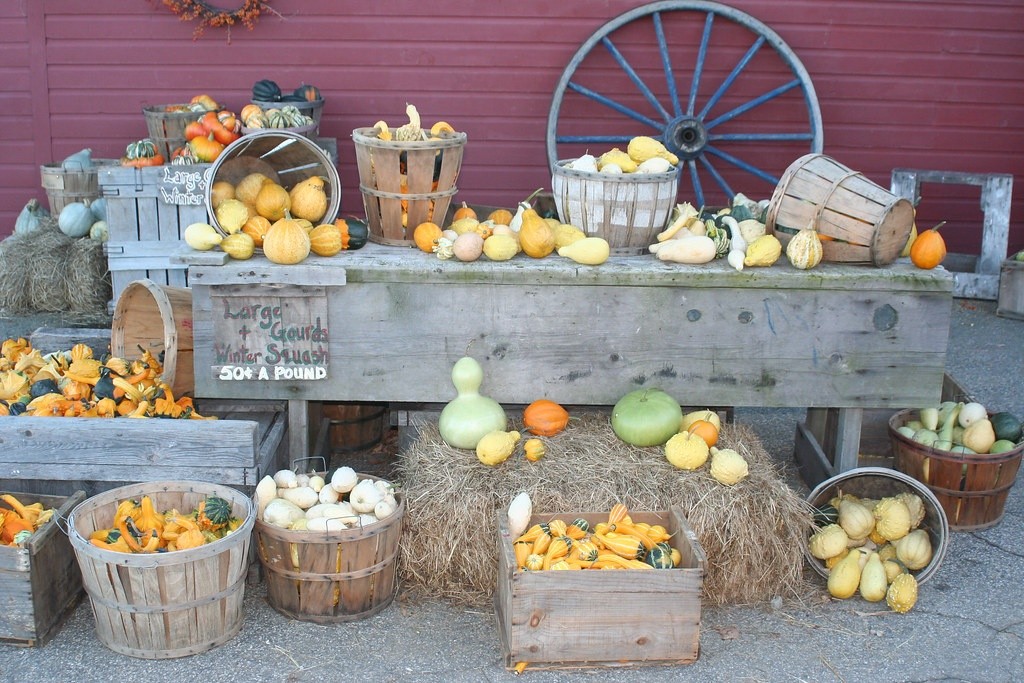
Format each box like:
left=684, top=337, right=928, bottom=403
left=1, top=78, right=1023, bottom=614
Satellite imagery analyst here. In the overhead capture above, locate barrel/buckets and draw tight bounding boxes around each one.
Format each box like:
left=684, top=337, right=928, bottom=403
left=142, top=103, right=226, bottom=163
left=250, top=97, right=325, bottom=136
left=39, top=158, right=120, bottom=217
left=766, top=153, right=914, bottom=269
left=254, top=455, right=406, bottom=626
left=322, top=400, right=384, bottom=451
left=241, top=123, right=316, bottom=143
left=802, top=467, right=948, bottom=586
left=52, top=482, right=259, bottom=658
left=352, top=127, right=466, bottom=248
left=887, top=407, right=1024, bottom=532
left=206, top=131, right=342, bottom=254
left=551, top=157, right=680, bottom=258
left=112, top=278, right=192, bottom=400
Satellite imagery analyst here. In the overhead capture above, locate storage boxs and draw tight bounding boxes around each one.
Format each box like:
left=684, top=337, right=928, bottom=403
left=798, top=467, right=951, bottom=598
left=51, top=480, right=260, bottom=660
left=318, top=402, right=388, bottom=450
left=494, top=505, right=709, bottom=673
left=551, top=154, right=680, bottom=260
left=996, top=250, right=1024, bottom=322
left=766, top=153, right=916, bottom=267
left=0, top=488, right=91, bottom=650
left=111, top=278, right=194, bottom=404
left=251, top=455, right=408, bottom=624
left=352, top=123, right=468, bottom=250
left=40, top=79, right=340, bottom=260
left=888, top=404, right=1024, bottom=531
